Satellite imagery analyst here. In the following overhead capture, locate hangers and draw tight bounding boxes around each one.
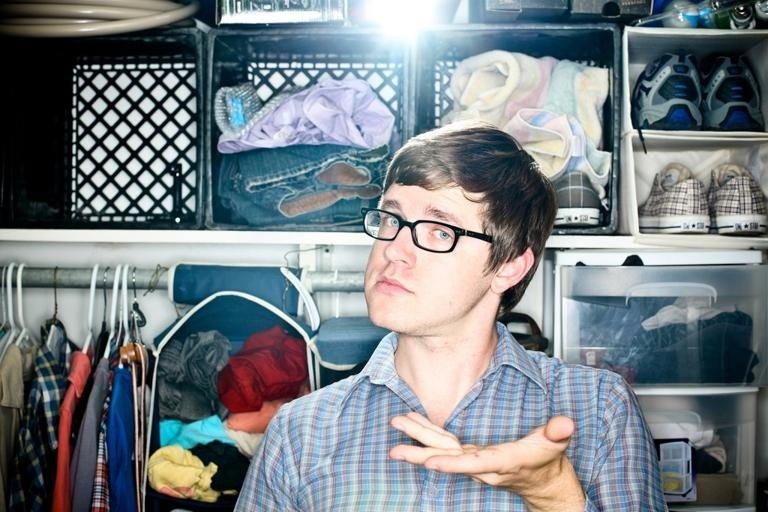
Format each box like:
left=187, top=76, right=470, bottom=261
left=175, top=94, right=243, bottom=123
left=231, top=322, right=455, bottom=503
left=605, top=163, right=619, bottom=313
left=0, top=260, right=144, bottom=371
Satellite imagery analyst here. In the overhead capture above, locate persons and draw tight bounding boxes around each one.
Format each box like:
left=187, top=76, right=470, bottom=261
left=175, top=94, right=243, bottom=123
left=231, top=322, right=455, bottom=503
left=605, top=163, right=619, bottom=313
left=231, top=121, right=670, bottom=511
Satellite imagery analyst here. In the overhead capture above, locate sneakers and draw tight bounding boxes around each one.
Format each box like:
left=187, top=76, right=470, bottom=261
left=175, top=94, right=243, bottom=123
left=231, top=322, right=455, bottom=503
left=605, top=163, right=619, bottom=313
left=701, top=49, right=765, bottom=134
left=710, top=161, right=768, bottom=234
left=551, top=170, right=606, bottom=227
left=636, top=162, right=711, bottom=235
left=630, top=47, right=704, bottom=133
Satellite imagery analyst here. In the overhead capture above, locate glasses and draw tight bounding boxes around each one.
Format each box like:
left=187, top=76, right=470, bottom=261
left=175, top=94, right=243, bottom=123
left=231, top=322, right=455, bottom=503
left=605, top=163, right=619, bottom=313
left=358, top=206, right=497, bottom=256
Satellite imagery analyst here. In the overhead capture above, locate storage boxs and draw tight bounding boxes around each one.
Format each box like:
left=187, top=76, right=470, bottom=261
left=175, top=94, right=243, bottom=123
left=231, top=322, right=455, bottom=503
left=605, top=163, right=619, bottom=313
left=631, top=388, right=759, bottom=512
left=558, top=264, right=768, bottom=388
